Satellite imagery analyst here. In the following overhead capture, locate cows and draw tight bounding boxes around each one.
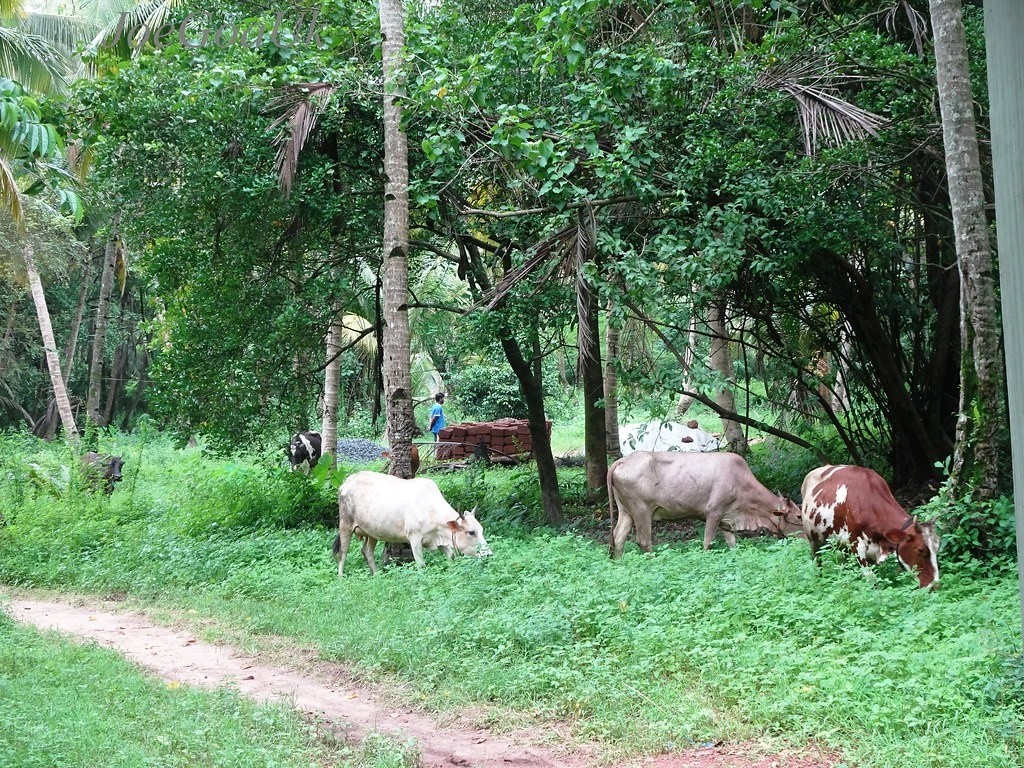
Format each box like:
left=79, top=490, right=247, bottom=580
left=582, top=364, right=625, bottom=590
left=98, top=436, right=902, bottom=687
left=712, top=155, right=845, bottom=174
left=287, top=431, right=322, bottom=474
left=336, top=470, right=492, bottom=579
left=605, top=451, right=808, bottom=561
left=801, top=463, right=941, bottom=592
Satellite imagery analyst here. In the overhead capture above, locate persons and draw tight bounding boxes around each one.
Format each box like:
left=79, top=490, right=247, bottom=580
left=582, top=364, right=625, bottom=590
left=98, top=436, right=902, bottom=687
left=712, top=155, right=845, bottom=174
left=426, top=393, right=445, bottom=442
